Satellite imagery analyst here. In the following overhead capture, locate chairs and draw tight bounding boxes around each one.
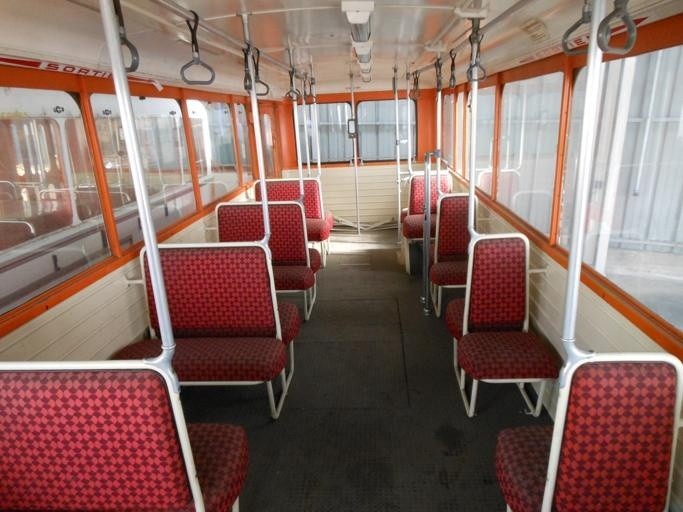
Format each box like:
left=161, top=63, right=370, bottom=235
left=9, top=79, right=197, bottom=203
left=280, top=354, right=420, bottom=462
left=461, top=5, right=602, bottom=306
left=0, top=360, right=250, bottom=512
left=401, top=172, right=453, bottom=276
left=494, top=353, right=683, bottom=512
left=252, top=177, right=334, bottom=268
left=428, top=192, right=479, bottom=319
left=214, top=201, right=317, bottom=322
left=109, top=242, right=301, bottom=420
left=446, top=233, right=559, bottom=418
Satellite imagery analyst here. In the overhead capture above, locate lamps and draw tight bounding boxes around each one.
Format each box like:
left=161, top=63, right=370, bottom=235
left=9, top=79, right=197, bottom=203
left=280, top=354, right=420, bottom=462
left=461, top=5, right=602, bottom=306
left=340, top=0, right=377, bottom=47
left=354, top=47, right=374, bottom=86
